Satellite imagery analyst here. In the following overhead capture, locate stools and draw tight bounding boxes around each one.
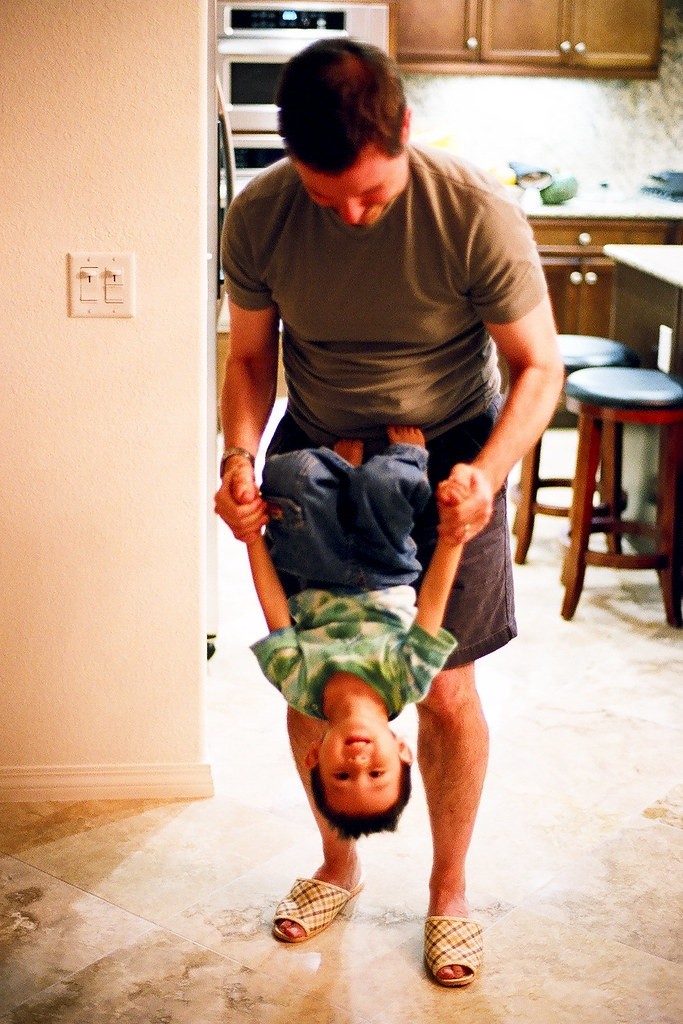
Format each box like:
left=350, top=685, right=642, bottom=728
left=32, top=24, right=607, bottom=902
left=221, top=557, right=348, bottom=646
left=562, top=364, right=683, bottom=628
left=512, top=333, right=628, bottom=565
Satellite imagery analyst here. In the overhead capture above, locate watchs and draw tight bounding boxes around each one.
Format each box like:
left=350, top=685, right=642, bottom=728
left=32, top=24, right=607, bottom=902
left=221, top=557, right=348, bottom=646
left=220, top=447, right=255, bottom=478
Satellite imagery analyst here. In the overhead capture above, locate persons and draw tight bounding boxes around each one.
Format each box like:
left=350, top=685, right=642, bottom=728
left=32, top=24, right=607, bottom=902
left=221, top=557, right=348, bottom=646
left=215, top=39, right=567, bottom=989
left=236, top=430, right=474, bottom=841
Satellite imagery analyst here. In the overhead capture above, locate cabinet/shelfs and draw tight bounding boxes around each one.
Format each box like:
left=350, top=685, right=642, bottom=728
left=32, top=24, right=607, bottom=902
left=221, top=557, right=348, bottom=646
left=512, top=218, right=683, bottom=431
left=478, top=0, right=662, bottom=81
left=389, top=0, right=479, bottom=76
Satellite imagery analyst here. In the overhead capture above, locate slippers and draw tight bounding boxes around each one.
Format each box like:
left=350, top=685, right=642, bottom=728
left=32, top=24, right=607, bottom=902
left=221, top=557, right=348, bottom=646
left=272, top=878, right=364, bottom=942
left=425, top=915, right=483, bottom=987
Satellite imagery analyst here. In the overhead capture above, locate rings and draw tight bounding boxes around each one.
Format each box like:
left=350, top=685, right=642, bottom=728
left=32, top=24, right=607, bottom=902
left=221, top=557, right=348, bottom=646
left=464, top=524, right=470, bottom=539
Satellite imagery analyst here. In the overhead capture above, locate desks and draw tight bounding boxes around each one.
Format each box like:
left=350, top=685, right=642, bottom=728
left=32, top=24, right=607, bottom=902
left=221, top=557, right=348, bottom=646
left=601, top=244, right=683, bottom=375
